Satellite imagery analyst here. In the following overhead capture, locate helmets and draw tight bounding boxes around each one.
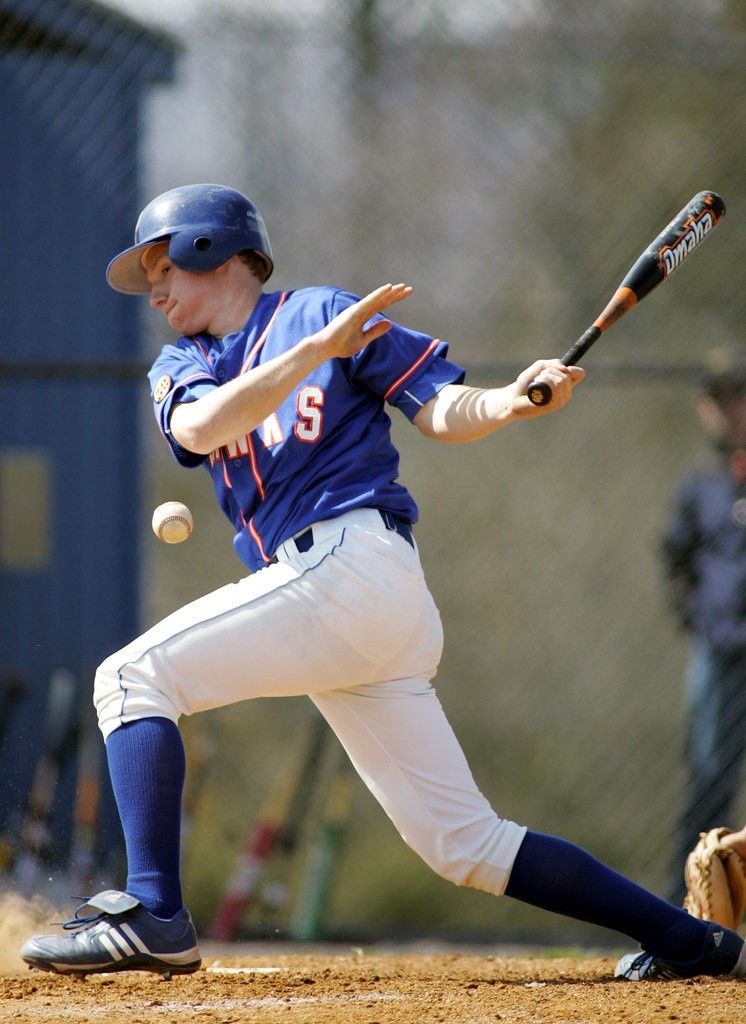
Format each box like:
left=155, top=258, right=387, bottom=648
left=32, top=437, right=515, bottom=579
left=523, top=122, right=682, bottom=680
left=106, top=184, right=275, bottom=296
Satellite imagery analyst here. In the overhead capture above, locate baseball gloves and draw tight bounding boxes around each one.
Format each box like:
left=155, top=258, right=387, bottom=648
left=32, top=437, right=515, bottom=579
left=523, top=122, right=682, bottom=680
left=681, top=825, right=746, bottom=931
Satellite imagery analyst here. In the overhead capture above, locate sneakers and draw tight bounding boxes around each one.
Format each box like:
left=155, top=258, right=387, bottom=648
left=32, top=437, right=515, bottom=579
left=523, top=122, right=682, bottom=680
left=615, top=922, right=746, bottom=979
left=20, top=893, right=202, bottom=973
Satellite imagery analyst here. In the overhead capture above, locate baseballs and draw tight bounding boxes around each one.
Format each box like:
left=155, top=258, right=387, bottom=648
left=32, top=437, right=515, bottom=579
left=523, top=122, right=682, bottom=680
left=152, top=501, right=193, bottom=544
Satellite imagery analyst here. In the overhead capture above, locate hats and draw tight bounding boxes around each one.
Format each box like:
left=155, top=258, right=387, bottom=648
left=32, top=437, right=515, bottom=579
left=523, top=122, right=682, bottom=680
left=702, top=344, right=745, bottom=385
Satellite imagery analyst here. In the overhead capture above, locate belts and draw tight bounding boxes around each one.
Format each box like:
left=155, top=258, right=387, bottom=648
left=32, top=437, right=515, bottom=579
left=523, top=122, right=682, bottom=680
left=266, top=517, right=414, bottom=565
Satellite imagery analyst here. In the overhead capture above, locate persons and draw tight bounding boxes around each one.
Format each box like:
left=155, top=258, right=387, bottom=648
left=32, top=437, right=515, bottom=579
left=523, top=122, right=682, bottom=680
left=20, top=183, right=746, bottom=983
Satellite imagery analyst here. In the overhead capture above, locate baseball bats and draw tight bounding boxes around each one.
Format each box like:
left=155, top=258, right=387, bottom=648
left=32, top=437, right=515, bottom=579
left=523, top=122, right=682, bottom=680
left=526, top=190, right=727, bottom=407
